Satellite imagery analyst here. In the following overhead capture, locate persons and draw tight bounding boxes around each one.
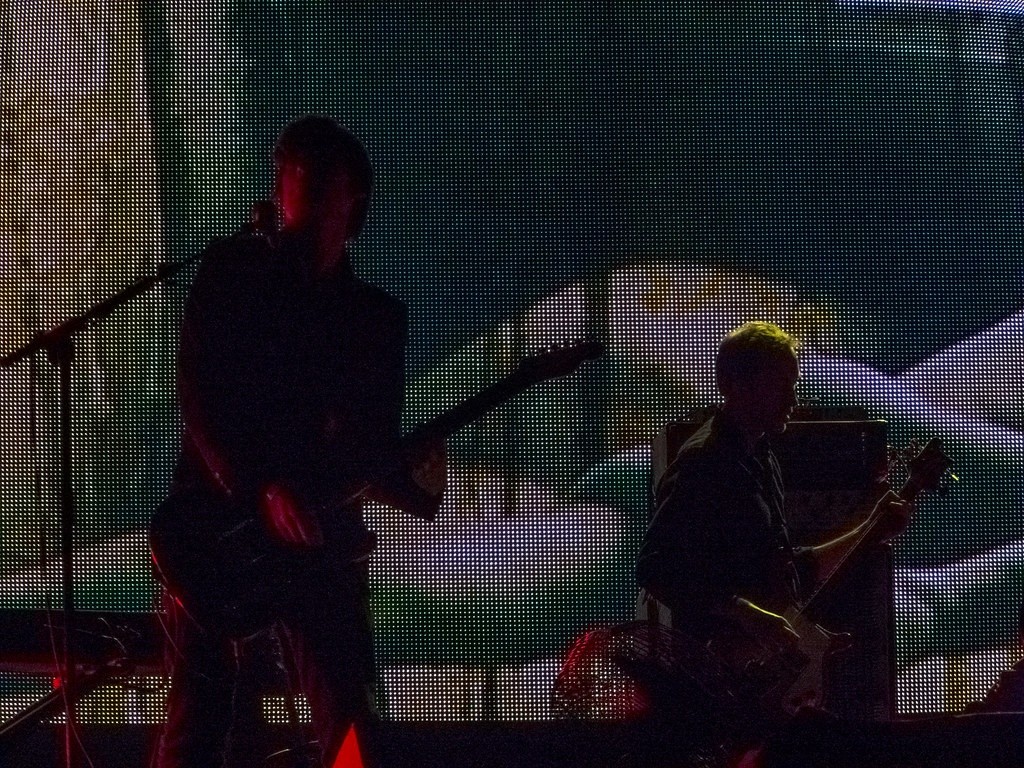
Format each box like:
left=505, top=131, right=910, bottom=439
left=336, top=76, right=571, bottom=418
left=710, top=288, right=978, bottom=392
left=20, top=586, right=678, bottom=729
left=636, top=321, right=918, bottom=768
left=146, top=113, right=454, bottom=768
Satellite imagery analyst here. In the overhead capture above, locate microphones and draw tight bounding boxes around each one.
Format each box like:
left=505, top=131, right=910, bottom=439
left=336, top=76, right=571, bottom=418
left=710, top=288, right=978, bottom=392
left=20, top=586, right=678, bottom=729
left=252, top=200, right=282, bottom=249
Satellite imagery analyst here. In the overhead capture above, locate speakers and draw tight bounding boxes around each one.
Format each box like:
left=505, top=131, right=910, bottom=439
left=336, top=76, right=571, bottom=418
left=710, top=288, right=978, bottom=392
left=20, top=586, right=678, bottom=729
left=791, top=538, right=895, bottom=721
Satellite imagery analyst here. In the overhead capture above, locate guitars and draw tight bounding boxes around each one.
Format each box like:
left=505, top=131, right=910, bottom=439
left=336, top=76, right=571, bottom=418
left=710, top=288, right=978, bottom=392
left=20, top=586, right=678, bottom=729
left=670, top=433, right=963, bottom=735
left=144, top=327, right=612, bottom=640
